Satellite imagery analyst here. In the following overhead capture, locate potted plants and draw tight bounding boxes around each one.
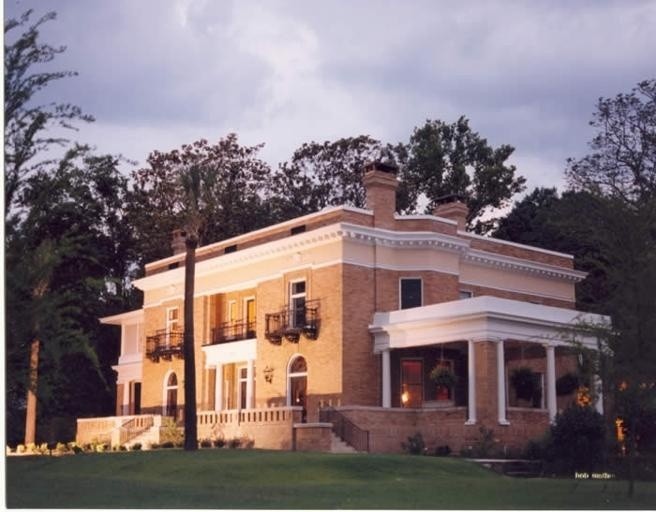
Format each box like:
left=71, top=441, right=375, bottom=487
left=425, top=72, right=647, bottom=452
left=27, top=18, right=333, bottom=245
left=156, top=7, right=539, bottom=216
left=430, top=366, right=455, bottom=400
left=511, top=367, right=534, bottom=407
